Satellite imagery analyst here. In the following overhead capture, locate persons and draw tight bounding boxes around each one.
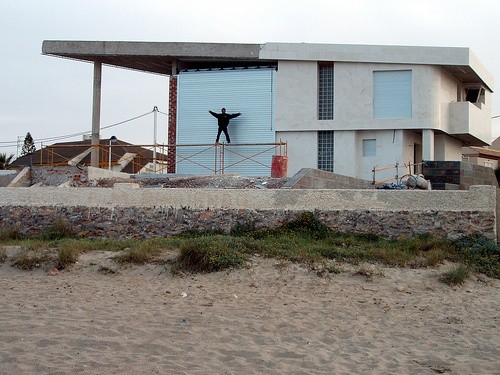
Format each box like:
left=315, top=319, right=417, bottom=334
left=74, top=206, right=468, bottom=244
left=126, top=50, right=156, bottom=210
left=208, top=108, right=242, bottom=143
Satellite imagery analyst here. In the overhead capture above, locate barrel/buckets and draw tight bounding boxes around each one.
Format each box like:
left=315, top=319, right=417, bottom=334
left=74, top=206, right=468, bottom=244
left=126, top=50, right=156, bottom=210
left=271, top=155, right=288, bottom=178
